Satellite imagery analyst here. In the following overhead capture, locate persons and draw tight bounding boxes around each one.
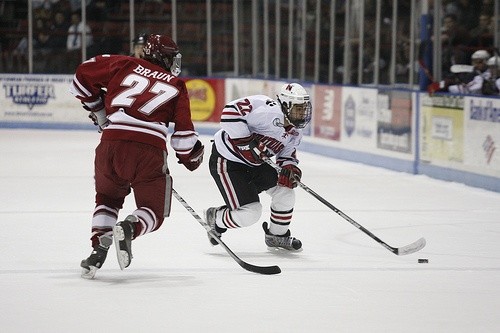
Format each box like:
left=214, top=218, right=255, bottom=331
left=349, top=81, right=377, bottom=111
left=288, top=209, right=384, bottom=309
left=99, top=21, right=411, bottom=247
left=203, top=83, right=312, bottom=250
left=0, top=0, right=133, bottom=75
left=69, top=31, right=205, bottom=272
left=285, top=1, right=500, bottom=98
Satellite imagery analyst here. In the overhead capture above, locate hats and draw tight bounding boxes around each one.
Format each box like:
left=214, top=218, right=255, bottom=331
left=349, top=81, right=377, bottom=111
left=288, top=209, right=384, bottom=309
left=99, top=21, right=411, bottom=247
left=472, top=50, right=490, bottom=59
left=486, top=55, right=500, bottom=66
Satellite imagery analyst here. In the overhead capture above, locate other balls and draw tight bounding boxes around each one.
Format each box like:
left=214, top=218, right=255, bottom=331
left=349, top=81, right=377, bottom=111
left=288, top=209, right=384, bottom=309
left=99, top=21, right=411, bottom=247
left=418, top=258, right=429, bottom=264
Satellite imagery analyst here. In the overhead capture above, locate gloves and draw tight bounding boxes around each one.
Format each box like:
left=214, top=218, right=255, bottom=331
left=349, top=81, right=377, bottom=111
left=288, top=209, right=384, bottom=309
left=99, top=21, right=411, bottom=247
left=277, top=164, right=302, bottom=189
left=228, top=134, right=265, bottom=167
left=88, top=102, right=112, bottom=133
left=175, top=139, right=204, bottom=171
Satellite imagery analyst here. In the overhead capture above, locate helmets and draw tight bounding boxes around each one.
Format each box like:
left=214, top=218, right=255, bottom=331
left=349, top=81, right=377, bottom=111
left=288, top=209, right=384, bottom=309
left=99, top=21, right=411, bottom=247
left=276, top=82, right=312, bottom=129
left=142, top=33, right=182, bottom=77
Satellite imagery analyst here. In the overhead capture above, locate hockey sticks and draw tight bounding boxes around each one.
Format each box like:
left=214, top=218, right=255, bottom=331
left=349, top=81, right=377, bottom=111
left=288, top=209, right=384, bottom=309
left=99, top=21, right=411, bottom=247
left=253, top=147, right=427, bottom=256
left=172, top=188, right=282, bottom=275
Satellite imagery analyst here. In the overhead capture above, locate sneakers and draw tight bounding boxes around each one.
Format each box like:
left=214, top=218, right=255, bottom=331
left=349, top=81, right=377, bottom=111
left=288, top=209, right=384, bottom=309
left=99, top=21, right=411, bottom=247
left=113, top=215, right=140, bottom=270
left=80, top=234, right=113, bottom=279
left=262, top=222, right=303, bottom=253
left=203, top=205, right=228, bottom=245
